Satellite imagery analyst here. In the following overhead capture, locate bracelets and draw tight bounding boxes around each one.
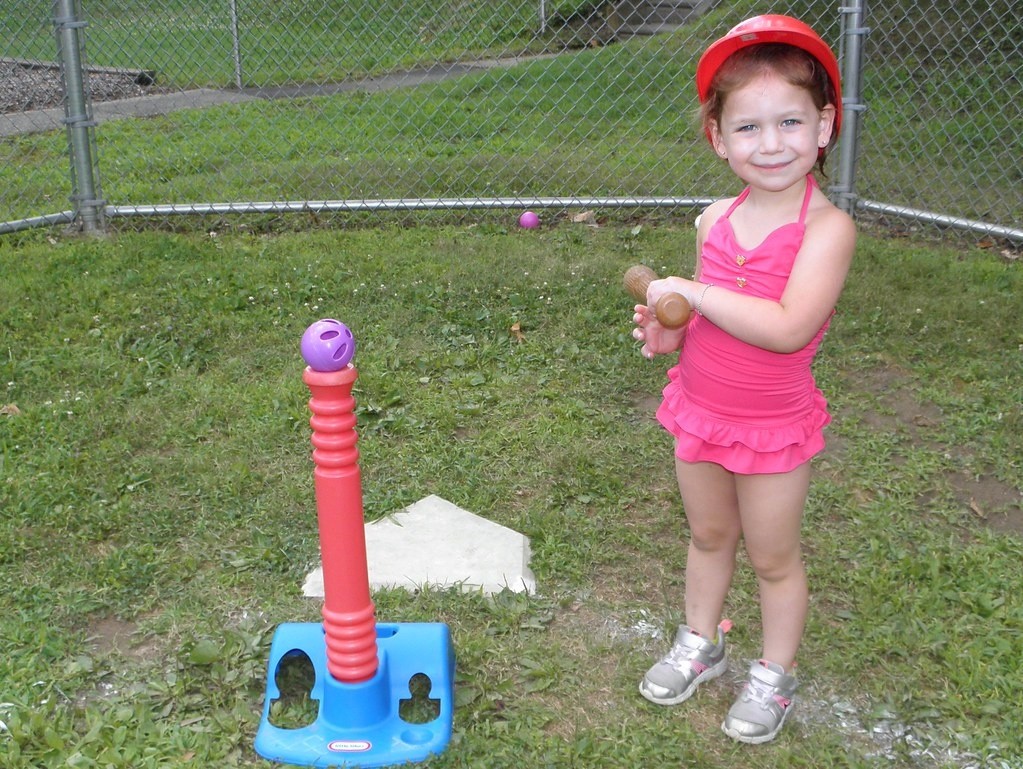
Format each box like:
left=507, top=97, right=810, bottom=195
left=698, top=284, right=714, bottom=313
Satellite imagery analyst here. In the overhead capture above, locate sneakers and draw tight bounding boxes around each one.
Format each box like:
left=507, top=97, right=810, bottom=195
left=639, top=619, right=732, bottom=705
left=721, top=659, right=799, bottom=744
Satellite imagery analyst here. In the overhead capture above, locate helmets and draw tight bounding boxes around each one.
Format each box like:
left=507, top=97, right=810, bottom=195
left=697, top=14, right=843, bottom=158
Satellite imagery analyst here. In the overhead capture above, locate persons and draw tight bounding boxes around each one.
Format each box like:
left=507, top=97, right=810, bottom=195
left=632, top=14, right=857, bottom=743
